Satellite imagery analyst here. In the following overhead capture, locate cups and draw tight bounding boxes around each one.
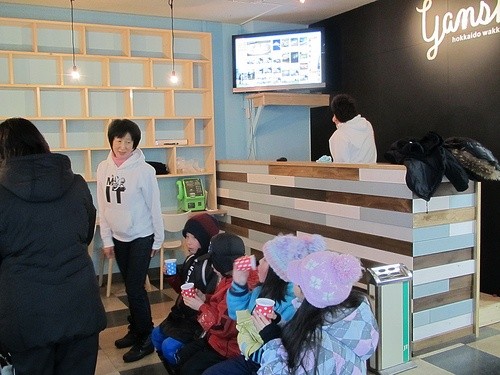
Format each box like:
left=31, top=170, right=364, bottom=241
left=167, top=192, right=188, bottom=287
left=163, top=259, right=177, bottom=276
left=180, top=282, right=196, bottom=300
left=254, top=298, right=275, bottom=323
left=234, top=254, right=256, bottom=272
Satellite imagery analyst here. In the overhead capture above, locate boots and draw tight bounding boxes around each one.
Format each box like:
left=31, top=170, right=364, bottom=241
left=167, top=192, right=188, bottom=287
left=115, top=315, right=137, bottom=348
left=123, top=322, right=155, bottom=363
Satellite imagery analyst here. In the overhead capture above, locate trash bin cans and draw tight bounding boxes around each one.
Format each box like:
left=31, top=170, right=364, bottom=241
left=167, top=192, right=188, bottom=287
left=366, top=263, right=418, bottom=375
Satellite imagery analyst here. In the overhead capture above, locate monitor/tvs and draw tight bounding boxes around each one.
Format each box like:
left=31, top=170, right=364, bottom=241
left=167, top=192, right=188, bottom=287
left=231, top=27, right=330, bottom=94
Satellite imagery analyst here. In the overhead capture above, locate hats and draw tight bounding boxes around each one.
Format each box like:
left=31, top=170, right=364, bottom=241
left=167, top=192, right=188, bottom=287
left=287, top=250, right=363, bottom=308
left=182, top=212, right=220, bottom=248
left=262, top=232, right=326, bottom=282
left=197, top=233, right=245, bottom=273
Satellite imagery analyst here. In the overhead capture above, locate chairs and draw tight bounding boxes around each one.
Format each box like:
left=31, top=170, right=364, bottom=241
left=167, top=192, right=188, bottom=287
left=88, top=210, right=192, bottom=297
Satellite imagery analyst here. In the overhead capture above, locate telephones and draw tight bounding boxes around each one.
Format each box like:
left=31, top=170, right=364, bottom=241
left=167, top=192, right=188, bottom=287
left=176, top=177, right=207, bottom=211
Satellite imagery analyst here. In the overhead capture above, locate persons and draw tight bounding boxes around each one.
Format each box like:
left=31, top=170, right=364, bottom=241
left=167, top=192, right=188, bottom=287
left=0, top=118, right=107, bottom=375
left=202, top=234, right=327, bottom=375
left=176, top=233, right=260, bottom=375
left=329, top=94, right=377, bottom=164
left=97, top=119, right=164, bottom=362
left=252, top=251, right=379, bottom=375
left=151, top=213, right=220, bottom=375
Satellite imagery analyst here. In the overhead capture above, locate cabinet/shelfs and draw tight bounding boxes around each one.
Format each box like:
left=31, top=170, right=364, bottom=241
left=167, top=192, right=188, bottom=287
left=0, top=18, right=217, bottom=214
